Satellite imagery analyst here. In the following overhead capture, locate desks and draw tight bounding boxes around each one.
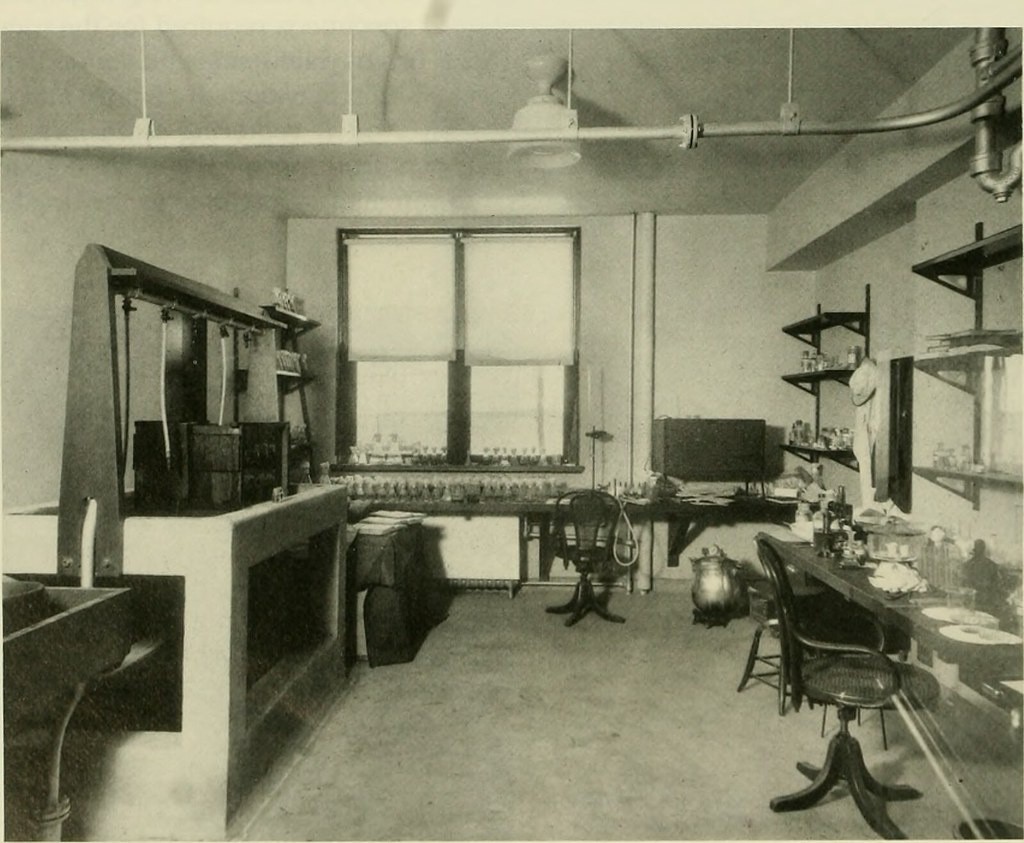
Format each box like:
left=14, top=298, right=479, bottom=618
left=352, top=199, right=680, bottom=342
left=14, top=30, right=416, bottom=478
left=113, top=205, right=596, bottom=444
left=357, top=495, right=800, bottom=566
left=755, top=532, right=1024, bottom=681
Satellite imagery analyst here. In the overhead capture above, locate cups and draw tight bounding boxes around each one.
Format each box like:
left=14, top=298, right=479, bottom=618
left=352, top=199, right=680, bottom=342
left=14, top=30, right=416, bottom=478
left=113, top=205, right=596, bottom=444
left=943, top=584, right=977, bottom=623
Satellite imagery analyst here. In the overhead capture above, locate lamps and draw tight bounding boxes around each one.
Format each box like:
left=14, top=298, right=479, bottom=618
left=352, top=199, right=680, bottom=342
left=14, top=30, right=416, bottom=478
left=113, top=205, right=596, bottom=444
left=502, top=52, right=583, bottom=166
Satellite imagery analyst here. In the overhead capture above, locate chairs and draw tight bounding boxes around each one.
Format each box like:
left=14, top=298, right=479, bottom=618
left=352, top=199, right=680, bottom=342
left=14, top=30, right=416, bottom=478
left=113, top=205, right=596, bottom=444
left=753, top=534, right=924, bottom=842
left=539, top=486, right=625, bottom=627
left=754, top=539, right=913, bottom=718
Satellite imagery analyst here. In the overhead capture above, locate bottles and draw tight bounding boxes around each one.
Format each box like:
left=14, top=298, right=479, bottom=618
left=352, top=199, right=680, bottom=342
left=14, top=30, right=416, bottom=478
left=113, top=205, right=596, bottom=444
left=933, top=441, right=973, bottom=475
left=469, top=444, right=562, bottom=467
left=299, top=459, right=563, bottom=506
left=346, top=430, right=447, bottom=465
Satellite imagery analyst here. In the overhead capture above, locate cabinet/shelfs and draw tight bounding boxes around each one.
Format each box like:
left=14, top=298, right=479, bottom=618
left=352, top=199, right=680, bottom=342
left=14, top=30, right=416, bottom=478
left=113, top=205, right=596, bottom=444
left=779, top=278, right=872, bottom=473
left=908, top=224, right=1023, bottom=513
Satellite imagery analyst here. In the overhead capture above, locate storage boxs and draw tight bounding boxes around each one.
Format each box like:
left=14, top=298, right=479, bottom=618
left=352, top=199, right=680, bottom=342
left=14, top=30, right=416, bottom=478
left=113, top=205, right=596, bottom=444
left=178, top=414, right=291, bottom=508
